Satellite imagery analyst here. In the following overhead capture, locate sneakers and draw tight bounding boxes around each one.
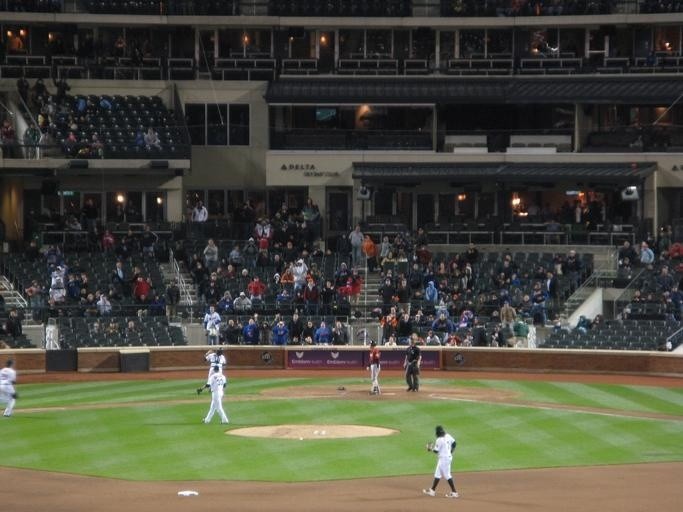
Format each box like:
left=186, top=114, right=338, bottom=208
left=445, top=492, right=458, bottom=498
left=407, top=388, right=418, bottom=392
left=423, top=488, right=436, bottom=497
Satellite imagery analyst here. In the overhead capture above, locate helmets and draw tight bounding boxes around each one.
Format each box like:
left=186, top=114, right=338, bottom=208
left=369, top=341, right=376, bottom=346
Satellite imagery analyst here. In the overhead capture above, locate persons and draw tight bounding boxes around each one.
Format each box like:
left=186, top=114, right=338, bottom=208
left=202, top=347, right=227, bottom=392
left=0, top=359, right=23, bottom=418
left=194, top=364, right=230, bottom=426
left=420, top=423, right=459, bottom=499
left=401, top=337, right=422, bottom=393
left=363, top=339, right=381, bottom=396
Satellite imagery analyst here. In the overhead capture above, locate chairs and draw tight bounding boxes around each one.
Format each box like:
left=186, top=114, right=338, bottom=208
left=380, top=250, right=595, bottom=346
left=36, top=92, right=184, bottom=153
left=1, top=241, right=186, bottom=349
left=187, top=242, right=353, bottom=346
left=538, top=259, right=683, bottom=351
